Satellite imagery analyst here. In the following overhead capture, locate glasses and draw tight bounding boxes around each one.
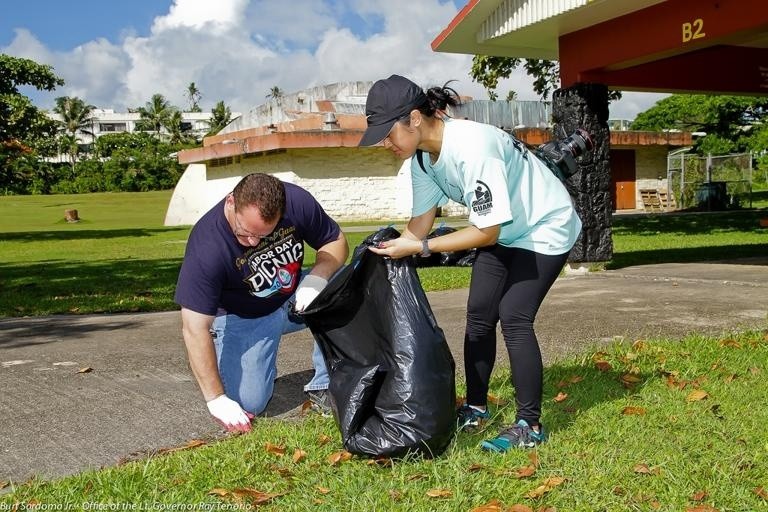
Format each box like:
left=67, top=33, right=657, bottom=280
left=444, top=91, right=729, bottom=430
left=235, top=229, right=277, bottom=242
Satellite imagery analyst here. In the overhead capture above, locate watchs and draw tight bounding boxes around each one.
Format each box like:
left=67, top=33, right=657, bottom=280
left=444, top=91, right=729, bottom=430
left=420, top=240, right=432, bottom=258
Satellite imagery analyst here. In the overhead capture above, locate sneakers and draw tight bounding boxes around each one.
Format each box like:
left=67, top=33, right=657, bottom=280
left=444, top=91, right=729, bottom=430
left=479, top=419, right=547, bottom=455
left=454, top=404, right=489, bottom=432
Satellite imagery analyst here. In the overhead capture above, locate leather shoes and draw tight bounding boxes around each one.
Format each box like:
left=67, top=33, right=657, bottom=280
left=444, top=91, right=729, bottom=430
left=307, top=389, right=332, bottom=412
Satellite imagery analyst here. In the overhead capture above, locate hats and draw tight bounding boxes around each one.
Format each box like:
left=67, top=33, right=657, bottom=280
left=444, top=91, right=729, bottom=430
left=356, top=74, right=427, bottom=149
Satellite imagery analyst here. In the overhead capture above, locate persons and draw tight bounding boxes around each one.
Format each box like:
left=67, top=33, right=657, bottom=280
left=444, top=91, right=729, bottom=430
left=174, top=170, right=349, bottom=432
left=358, top=74, right=582, bottom=455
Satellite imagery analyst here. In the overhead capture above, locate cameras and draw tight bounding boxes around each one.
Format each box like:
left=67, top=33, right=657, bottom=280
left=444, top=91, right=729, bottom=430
left=536, top=128, right=595, bottom=182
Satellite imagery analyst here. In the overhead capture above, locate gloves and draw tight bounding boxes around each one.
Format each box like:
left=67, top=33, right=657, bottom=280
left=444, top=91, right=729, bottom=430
left=294, top=274, right=329, bottom=311
left=206, top=393, right=254, bottom=433
